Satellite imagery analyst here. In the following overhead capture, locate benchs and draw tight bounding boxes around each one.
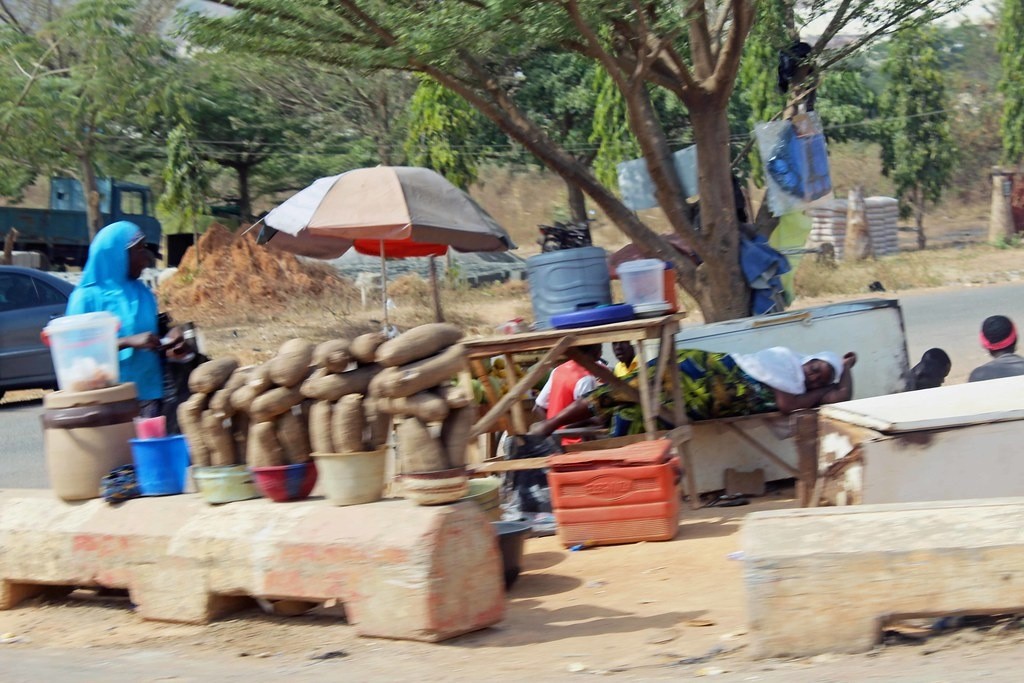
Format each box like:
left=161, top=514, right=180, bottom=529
left=738, top=496, right=1024, bottom=661
left=683, top=406, right=826, bottom=507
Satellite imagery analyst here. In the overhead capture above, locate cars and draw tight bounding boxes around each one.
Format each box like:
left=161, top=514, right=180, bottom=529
left=0, top=264, right=210, bottom=411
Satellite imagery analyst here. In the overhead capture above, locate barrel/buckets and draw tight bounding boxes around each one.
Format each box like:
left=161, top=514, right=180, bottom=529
left=527, top=245, right=611, bottom=329
left=615, top=259, right=667, bottom=307
left=664, top=262, right=678, bottom=313
left=42, top=381, right=140, bottom=502
left=39, top=311, right=122, bottom=391
left=125, top=433, right=189, bottom=495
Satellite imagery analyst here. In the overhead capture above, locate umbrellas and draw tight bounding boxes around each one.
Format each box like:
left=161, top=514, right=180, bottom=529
left=241, top=165, right=518, bottom=337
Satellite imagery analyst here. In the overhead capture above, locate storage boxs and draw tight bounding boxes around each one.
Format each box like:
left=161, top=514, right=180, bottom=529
left=548, top=438, right=685, bottom=549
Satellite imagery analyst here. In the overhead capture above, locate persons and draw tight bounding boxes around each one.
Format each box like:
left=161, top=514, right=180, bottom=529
left=531, top=344, right=612, bottom=447
left=968, top=315, right=1024, bottom=382
left=613, top=340, right=637, bottom=377
left=910, top=349, right=951, bottom=390
left=65, top=221, right=189, bottom=420
left=526, top=346, right=856, bottom=437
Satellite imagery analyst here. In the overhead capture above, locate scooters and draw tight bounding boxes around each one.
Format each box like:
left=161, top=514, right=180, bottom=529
left=535, top=209, right=598, bottom=254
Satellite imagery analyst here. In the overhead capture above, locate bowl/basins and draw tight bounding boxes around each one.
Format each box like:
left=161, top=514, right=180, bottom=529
left=251, top=458, right=317, bottom=504
left=309, top=445, right=388, bottom=506
left=460, top=479, right=503, bottom=520
left=395, top=464, right=473, bottom=505
left=491, top=521, right=535, bottom=592
left=189, top=465, right=266, bottom=503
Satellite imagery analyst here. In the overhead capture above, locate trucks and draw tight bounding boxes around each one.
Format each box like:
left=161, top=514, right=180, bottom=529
left=0, top=175, right=165, bottom=272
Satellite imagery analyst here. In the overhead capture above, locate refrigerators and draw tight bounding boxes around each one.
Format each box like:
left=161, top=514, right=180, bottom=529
left=603, top=294, right=919, bottom=499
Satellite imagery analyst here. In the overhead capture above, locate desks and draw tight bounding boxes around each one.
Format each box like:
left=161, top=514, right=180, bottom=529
left=464, top=311, right=700, bottom=510
